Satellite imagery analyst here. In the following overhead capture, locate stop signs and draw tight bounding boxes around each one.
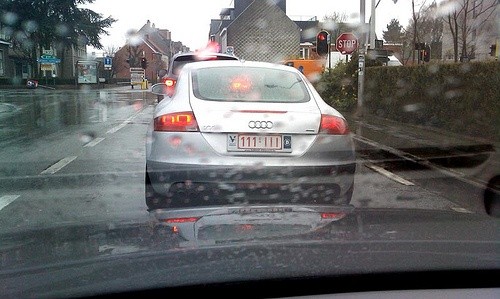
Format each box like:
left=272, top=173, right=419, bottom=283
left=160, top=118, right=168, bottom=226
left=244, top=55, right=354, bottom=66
left=336, top=33, right=358, bottom=55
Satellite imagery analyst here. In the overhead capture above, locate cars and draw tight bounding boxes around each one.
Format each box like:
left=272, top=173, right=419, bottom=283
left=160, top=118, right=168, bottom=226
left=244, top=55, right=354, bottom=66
left=153, top=52, right=240, bottom=105
left=144, top=59, right=356, bottom=205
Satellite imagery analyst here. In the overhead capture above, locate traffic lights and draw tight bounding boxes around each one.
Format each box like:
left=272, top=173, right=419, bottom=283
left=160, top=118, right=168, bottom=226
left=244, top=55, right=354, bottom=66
left=317, top=30, right=329, bottom=58
left=489, top=44, right=496, bottom=57
